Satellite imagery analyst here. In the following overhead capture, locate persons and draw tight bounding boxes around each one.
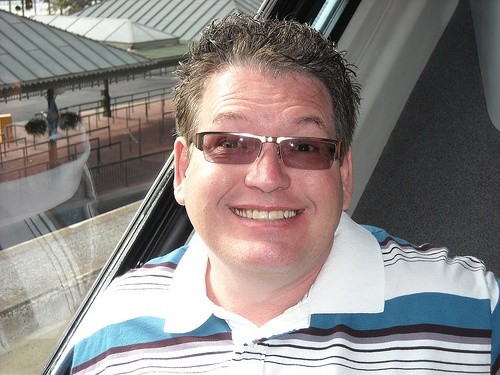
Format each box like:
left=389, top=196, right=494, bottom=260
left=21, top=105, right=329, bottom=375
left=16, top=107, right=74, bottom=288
left=51, top=16, right=500, bottom=374
left=0, top=98, right=95, bottom=357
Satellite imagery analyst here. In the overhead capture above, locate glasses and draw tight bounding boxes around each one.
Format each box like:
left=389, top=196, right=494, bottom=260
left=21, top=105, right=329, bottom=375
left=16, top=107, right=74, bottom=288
left=189, top=131, right=342, bottom=171
